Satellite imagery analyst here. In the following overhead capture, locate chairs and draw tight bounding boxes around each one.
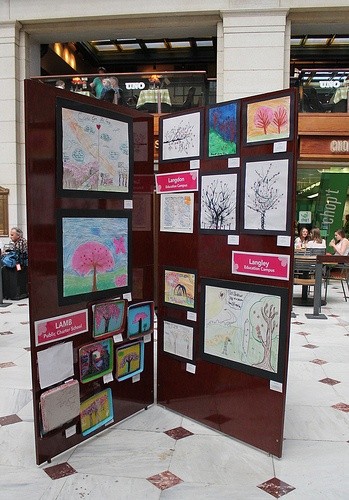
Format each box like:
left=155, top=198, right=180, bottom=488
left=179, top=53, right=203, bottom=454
left=293, top=244, right=349, bottom=307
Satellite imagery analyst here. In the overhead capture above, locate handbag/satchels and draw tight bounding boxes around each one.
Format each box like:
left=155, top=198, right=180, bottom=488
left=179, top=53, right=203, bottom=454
left=2, top=248, right=27, bottom=267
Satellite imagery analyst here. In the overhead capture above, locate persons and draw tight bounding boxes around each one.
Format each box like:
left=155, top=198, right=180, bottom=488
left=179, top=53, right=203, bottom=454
left=294, top=227, right=326, bottom=279
left=323, top=230, right=349, bottom=276
left=90, top=67, right=121, bottom=104
left=303, top=76, right=333, bottom=112
left=344, top=214, right=349, bottom=238
left=2, top=226, right=28, bottom=265
left=55, top=80, right=65, bottom=89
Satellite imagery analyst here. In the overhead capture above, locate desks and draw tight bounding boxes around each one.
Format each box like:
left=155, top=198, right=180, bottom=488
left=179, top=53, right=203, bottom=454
left=293, top=255, right=337, bottom=307
left=136, top=89, right=172, bottom=113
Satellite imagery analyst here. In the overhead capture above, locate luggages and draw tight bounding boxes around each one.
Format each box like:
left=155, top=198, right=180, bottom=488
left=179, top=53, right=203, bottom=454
left=2, top=238, right=28, bottom=300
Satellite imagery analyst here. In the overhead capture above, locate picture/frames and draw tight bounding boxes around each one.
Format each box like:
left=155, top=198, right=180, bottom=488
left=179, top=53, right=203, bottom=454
left=162, top=266, right=197, bottom=311
left=158, top=190, right=195, bottom=235
left=76, top=338, right=114, bottom=387
left=200, top=276, right=289, bottom=384
left=239, top=152, right=294, bottom=237
left=195, top=168, right=240, bottom=235
left=127, top=300, right=156, bottom=340
left=159, top=107, right=204, bottom=165
left=37, top=380, right=82, bottom=439
left=92, top=296, right=128, bottom=339
left=241, top=91, right=294, bottom=148
left=158, top=315, right=196, bottom=364
left=53, top=206, right=133, bottom=307
left=115, top=339, right=145, bottom=382
left=55, top=96, right=134, bottom=203
left=76, top=387, right=115, bottom=437
left=203, top=99, right=241, bottom=162
